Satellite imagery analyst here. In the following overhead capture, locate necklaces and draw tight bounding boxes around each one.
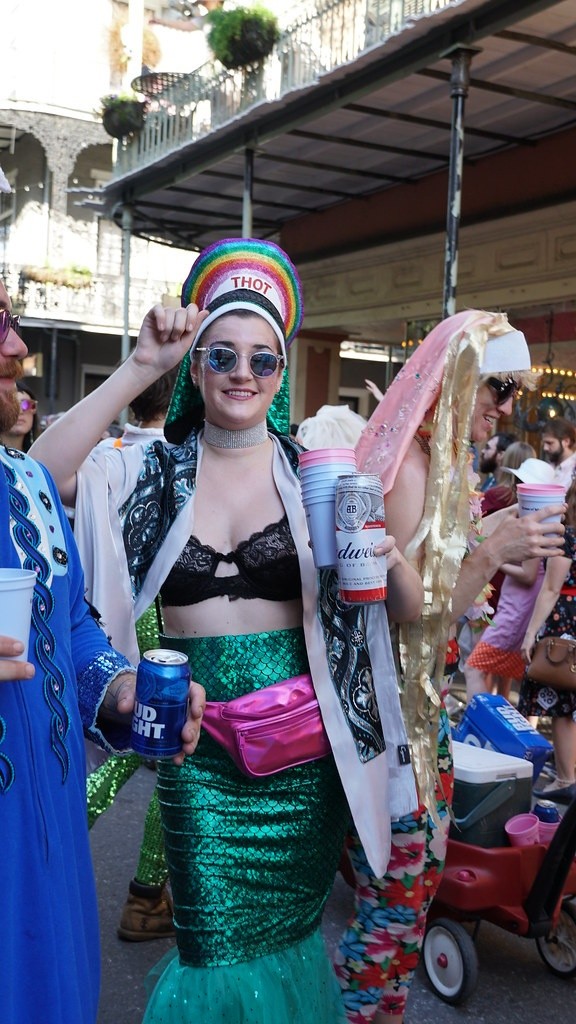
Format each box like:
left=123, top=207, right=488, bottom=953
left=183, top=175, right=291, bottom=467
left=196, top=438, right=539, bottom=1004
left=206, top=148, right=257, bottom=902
left=204, top=418, right=268, bottom=449
left=414, top=433, right=431, bottom=455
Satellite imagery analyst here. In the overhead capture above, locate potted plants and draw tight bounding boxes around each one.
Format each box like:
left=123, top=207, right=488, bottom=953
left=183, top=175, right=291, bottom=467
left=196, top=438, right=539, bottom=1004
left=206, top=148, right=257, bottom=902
left=203, top=2, right=279, bottom=70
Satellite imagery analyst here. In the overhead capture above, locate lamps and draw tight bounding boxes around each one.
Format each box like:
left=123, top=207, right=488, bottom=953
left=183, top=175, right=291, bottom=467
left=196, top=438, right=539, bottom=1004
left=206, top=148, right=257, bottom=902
left=401, top=331, right=414, bottom=348
left=417, top=328, right=426, bottom=345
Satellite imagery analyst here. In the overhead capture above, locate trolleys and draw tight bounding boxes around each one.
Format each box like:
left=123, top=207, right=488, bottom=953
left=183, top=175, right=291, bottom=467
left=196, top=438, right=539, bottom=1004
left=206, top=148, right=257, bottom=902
left=337, top=799, right=576, bottom=1008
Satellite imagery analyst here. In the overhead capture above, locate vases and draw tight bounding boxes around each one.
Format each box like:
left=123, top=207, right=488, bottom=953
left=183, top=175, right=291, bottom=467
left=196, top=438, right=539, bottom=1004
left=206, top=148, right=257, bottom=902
left=102, top=101, right=146, bottom=141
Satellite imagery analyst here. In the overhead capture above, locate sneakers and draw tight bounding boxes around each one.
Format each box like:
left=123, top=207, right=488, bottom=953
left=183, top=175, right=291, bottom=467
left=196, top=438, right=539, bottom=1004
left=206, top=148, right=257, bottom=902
left=118, top=876, right=175, bottom=940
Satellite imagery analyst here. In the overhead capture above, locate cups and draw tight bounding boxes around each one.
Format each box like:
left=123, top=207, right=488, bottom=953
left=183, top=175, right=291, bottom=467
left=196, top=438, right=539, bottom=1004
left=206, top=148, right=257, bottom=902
left=297, top=448, right=359, bottom=570
left=516, top=483, right=567, bottom=552
left=530, top=811, right=562, bottom=843
left=505, top=814, right=541, bottom=846
left=0, top=567, right=38, bottom=663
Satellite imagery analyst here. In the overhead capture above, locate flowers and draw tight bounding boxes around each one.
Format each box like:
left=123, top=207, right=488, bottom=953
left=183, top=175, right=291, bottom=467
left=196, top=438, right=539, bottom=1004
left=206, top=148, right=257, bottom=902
left=92, top=86, right=140, bottom=120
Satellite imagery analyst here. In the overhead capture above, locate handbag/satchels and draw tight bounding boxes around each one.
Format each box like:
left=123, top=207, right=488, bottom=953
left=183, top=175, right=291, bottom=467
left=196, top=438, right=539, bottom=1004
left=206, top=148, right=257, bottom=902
left=202, top=673, right=331, bottom=777
left=528, top=636, right=576, bottom=688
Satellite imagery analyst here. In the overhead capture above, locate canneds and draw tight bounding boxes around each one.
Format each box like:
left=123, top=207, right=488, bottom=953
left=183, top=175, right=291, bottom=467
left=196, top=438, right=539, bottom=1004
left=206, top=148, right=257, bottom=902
left=131, top=649, right=193, bottom=759
left=534, top=799, right=559, bottom=824
left=335, top=472, right=388, bottom=603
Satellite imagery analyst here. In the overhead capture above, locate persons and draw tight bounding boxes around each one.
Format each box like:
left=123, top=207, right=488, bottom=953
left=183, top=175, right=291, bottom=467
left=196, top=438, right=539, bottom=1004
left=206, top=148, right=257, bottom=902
left=0, top=274, right=204, bottom=1024
left=288, top=424, right=299, bottom=439
left=5, top=386, right=41, bottom=450
left=25, top=239, right=423, bottom=1024
left=479, top=439, right=537, bottom=706
left=365, top=378, right=382, bottom=405
left=527, top=415, right=576, bottom=806
left=98, top=346, right=181, bottom=445
left=333, top=305, right=545, bottom=1024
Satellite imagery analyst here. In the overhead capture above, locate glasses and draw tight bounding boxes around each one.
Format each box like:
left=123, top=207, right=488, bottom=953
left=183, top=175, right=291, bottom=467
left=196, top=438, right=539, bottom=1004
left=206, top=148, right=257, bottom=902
left=487, top=377, right=523, bottom=406
left=196, top=347, right=284, bottom=375
left=19, top=399, right=35, bottom=411
left=0, top=308, right=20, bottom=344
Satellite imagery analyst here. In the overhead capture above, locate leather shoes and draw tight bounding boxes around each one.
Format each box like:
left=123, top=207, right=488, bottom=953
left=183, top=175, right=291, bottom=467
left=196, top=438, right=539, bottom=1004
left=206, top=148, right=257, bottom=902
left=532, top=783, right=576, bottom=800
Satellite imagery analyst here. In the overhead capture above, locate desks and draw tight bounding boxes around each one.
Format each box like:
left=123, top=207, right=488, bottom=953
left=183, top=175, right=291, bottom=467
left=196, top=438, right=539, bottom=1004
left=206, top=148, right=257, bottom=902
left=131, top=71, right=208, bottom=136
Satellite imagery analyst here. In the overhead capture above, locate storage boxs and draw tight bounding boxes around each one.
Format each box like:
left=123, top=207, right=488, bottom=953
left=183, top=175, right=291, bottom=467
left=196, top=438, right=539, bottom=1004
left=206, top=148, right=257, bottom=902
left=450, top=692, right=555, bottom=788
left=450, top=738, right=534, bottom=848
